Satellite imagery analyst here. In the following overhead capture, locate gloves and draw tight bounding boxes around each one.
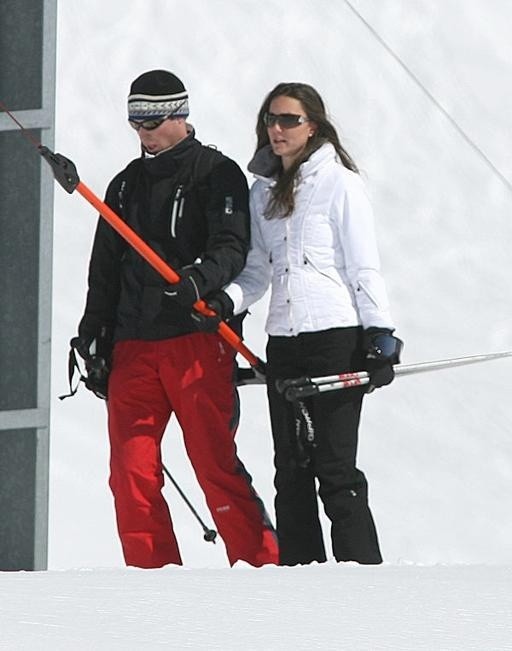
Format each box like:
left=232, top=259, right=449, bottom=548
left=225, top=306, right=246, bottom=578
left=353, top=328, right=402, bottom=392
left=191, top=289, right=235, bottom=334
left=161, top=256, right=221, bottom=308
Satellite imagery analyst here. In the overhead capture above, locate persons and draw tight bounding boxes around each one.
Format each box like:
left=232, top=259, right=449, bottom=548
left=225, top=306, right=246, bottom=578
left=188, top=83, right=405, bottom=564
left=78, top=70, right=280, bottom=569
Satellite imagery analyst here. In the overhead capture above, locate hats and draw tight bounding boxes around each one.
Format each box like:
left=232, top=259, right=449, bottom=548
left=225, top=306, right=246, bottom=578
left=125, top=69, right=189, bottom=119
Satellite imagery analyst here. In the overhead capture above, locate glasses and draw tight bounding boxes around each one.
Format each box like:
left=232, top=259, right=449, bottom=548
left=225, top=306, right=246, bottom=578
left=127, top=102, right=184, bottom=130
left=262, top=112, right=311, bottom=129
